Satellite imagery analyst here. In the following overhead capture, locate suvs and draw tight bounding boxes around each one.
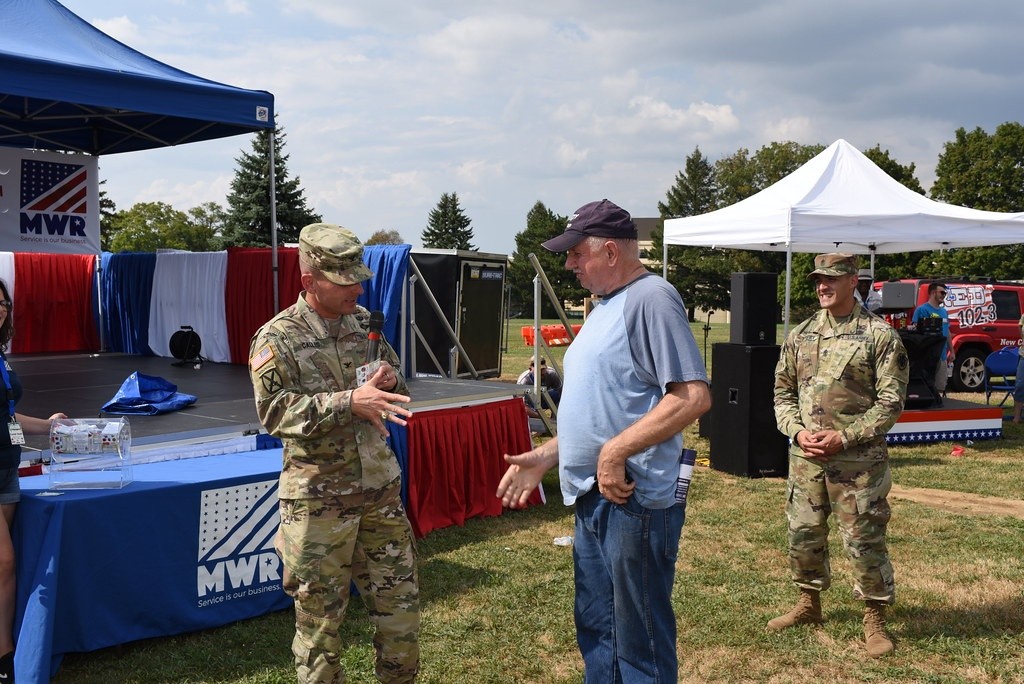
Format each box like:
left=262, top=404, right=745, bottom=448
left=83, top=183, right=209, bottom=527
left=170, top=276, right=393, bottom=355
left=874, top=276, right=1024, bottom=393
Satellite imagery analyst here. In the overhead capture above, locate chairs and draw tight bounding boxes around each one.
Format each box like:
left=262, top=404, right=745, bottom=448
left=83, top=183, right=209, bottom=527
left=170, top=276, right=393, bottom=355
left=982, top=346, right=1023, bottom=415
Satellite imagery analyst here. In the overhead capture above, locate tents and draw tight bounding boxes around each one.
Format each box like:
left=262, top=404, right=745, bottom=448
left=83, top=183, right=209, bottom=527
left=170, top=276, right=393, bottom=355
left=0, top=0, right=280, bottom=361
left=662, top=138, right=1024, bottom=344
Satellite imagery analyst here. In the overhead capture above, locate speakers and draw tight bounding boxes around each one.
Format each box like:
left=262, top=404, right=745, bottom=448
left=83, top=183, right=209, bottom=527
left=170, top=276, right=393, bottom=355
left=700, top=272, right=790, bottom=479
left=905, top=377, right=935, bottom=409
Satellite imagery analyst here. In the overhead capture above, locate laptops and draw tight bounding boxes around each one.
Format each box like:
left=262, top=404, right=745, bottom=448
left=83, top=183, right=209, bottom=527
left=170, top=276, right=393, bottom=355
left=872, top=283, right=915, bottom=315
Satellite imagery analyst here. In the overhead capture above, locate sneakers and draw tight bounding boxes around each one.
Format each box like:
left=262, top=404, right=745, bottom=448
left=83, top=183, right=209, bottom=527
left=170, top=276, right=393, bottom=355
left=0, top=651, right=15, bottom=684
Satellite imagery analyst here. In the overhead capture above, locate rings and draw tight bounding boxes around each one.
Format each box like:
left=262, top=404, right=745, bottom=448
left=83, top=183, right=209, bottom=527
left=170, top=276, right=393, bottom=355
left=381, top=411, right=391, bottom=420
left=386, top=373, right=391, bottom=380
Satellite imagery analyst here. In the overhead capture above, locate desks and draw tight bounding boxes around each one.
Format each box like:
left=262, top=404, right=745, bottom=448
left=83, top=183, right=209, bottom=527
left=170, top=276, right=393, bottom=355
left=19, top=447, right=283, bottom=684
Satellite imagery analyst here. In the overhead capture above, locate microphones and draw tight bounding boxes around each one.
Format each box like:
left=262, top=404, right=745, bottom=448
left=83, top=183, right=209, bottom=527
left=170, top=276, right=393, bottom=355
left=365, top=311, right=384, bottom=365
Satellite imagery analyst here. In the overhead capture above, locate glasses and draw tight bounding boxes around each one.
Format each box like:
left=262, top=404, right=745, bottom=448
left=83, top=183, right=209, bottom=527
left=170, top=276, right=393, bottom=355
left=0, top=299, right=12, bottom=312
left=532, top=360, right=545, bottom=367
left=815, top=274, right=850, bottom=285
left=935, top=291, right=947, bottom=296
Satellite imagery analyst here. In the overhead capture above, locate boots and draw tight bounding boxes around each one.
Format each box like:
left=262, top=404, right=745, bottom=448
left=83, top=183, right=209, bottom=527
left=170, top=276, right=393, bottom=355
left=767, top=588, right=822, bottom=631
left=862, top=601, right=893, bottom=659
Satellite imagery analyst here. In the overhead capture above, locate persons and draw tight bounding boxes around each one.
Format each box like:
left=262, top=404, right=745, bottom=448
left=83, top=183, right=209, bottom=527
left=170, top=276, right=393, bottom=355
left=1013, top=313, right=1024, bottom=424
left=516, top=354, right=563, bottom=421
left=767, top=252, right=910, bottom=657
left=495, top=199, right=712, bottom=684
left=854, top=269, right=901, bottom=320
left=247, top=223, right=421, bottom=684
left=911, top=282, right=955, bottom=398
left=0, top=278, right=74, bottom=684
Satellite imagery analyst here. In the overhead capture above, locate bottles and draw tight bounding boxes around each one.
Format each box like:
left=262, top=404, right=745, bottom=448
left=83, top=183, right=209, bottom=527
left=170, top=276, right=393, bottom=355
left=552, top=537, right=574, bottom=546
left=674, top=449, right=698, bottom=502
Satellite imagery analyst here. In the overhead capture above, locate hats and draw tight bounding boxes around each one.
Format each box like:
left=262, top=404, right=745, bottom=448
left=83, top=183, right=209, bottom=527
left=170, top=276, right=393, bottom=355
left=541, top=199, right=638, bottom=252
left=857, top=269, right=874, bottom=280
left=529, top=355, right=547, bottom=370
left=807, top=253, right=862, bottom=282
left=299, top=223, right=375, bottom=286
